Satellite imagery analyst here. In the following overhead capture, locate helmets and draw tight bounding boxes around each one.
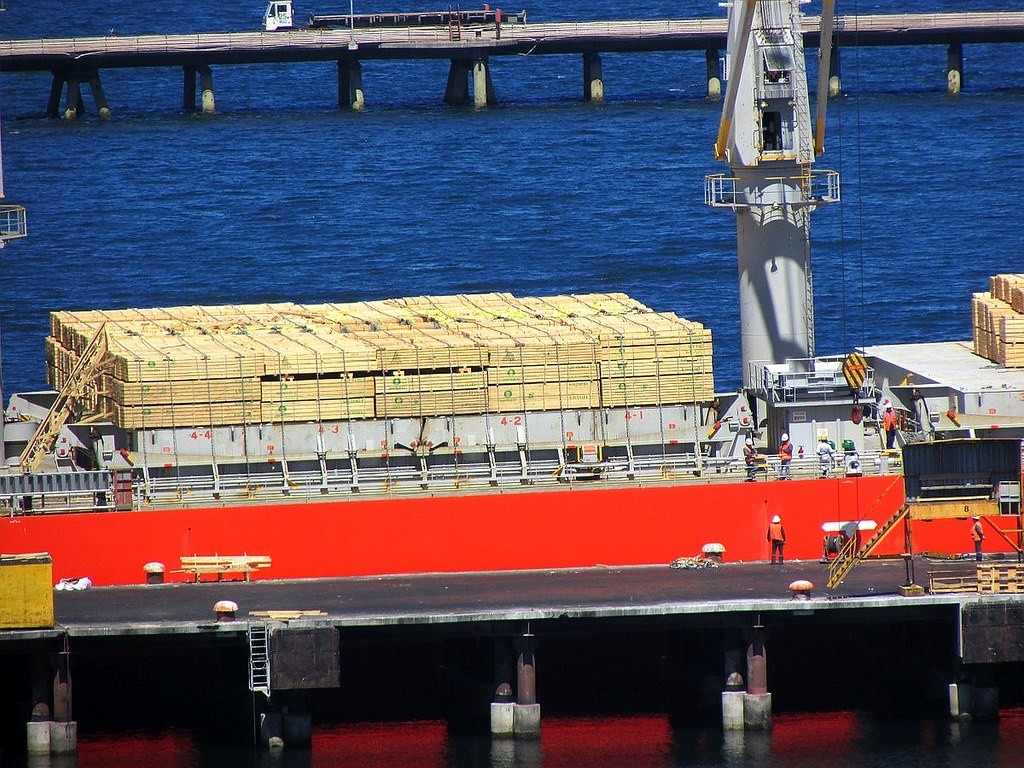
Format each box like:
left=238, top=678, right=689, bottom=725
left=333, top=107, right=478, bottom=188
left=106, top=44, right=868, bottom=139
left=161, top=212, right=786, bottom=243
left=745, top=438, right=753, bottom=445
left=771, top=514, right=781, bottom=523
left=972, top=515, right=981, bottom=520
left=820, top=435, right=827, bottom=440
left=884, top=402, right=893, bottom=409
left=781, top=433, right=788, bottom=442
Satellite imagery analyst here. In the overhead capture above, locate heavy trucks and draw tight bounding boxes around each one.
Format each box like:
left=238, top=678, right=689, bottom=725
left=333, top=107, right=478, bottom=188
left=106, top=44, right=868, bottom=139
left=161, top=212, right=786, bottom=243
left=261, top=1, right=527, bottom=32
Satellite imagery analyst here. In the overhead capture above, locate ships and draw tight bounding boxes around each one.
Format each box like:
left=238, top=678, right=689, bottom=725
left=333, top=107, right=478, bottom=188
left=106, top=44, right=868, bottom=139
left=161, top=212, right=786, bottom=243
left=5, top=0, right=1023, bottom=765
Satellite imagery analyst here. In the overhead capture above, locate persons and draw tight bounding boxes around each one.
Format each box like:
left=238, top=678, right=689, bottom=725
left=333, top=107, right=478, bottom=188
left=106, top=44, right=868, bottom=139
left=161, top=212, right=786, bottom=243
left=817, top=435, right=837, bottom=477
left=971, top=516, right=986, bottom=561
left=882, top=403, right=897, bottom=449
left=706, top=399, right=721, bottom=425
left=767, top=515, right=786, bottom=565
left=779, top=433, right=793, bottom=481
left=743, top=438, right=759, bottom=478
